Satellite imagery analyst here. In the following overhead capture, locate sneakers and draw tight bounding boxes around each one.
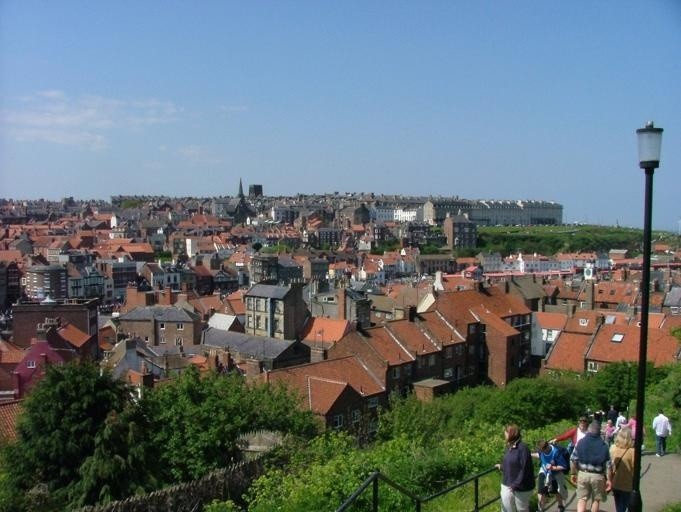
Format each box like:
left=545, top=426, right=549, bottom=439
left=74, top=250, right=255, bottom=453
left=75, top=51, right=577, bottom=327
left=656, top=453, right=661, bottom=457
left=558, top=505, right=564, bottom=512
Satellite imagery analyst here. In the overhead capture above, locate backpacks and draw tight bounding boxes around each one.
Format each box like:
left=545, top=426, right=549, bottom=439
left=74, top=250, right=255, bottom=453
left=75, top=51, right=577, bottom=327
left=555, top=444, right=570, bottom=465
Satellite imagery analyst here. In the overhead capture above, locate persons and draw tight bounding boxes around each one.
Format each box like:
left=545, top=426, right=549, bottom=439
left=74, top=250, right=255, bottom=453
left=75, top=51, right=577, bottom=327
left=569, top=422, right=613, bottom=512
left=619, top=415, right=645, bottom=455
left=549, top=415, right=591, bottom=451
left=611, top=428, right=634, bottom=512
left=494, top=425, right=535, bottom=512
left=530, top=440, right=570, bottom=512
left=652, top=408, right=672, bottom=457
left=586, top=404, right=627, bottom=446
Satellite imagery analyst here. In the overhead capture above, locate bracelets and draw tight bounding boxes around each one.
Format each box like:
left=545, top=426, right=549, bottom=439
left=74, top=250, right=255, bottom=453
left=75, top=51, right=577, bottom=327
left=570, top=473, right=577, bottom=476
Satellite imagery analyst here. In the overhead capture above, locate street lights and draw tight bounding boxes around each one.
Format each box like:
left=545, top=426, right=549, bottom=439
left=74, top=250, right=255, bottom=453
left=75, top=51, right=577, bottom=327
left=627, top=120, right=664, bottom=511
